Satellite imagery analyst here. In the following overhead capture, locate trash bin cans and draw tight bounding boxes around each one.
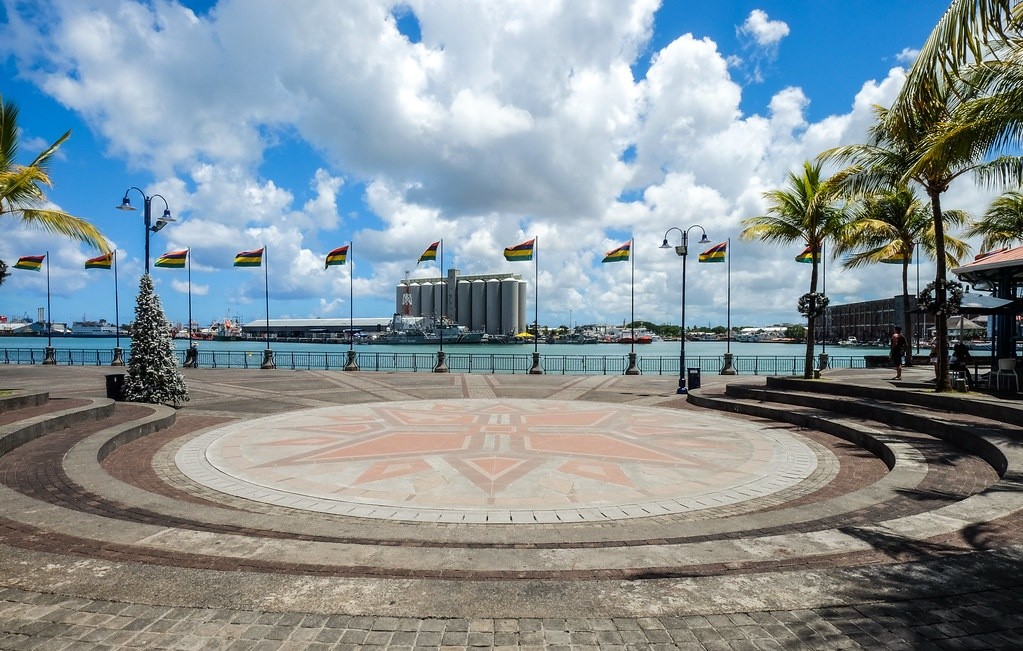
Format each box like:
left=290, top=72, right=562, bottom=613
left=105, top=374, right=125, bottom=401
left=687, top=368, right=700, bottom=390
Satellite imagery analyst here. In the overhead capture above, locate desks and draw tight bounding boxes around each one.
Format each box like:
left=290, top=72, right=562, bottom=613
left=965, top=356, right=997, bottom=392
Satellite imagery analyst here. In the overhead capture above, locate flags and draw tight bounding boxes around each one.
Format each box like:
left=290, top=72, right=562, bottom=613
left=794, top=243, right=821, bottom=263
left=601, top=240, right=631, bottom=262
left=153, top=249, right=188, bottom=268
left=84, top=252, right=113, bottom=269
left=233, top=248, right=263, bottom=266
left=12, top=254, right=45, bottom=271
left=417, top=242, right=439, bottom=263
left=324, top=245, right=348, bottom=269
left=503, top=238, right=535, bottom=261
left=698, top=241, right=727, bottom=262
left=879, top=243, right=914, bottom=264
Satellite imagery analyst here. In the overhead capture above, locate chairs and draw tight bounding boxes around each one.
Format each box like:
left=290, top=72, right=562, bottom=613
left=939, top=354, right=1019, bottom=391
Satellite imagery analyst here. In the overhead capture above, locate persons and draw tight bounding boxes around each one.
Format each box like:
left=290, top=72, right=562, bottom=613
left=890, top=325, right=908, bottom=380
left=948, top=344, right=974, bottom=389
left=930, top=337, right=938, bottom=378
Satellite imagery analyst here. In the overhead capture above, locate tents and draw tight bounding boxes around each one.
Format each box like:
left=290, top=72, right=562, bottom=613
left=930, top=316, right=983, bottom=330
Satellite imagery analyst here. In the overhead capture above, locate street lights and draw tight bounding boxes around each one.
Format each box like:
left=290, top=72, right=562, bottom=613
left=659, top=226, right=711, bottom=394
left=115, top=187, right=176, bottom=274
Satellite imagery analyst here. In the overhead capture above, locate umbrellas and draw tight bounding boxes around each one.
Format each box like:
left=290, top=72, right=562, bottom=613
left=903, top=285, right=1013, bottom=344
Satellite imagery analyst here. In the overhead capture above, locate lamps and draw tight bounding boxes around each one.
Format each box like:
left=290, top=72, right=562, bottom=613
left=973, top=273, right=997, bottom=291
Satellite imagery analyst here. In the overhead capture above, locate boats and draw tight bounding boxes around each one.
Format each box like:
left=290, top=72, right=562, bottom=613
left=366, top=269, right=484, bottom=344
left=171, top=309, right=246, bottom=341
